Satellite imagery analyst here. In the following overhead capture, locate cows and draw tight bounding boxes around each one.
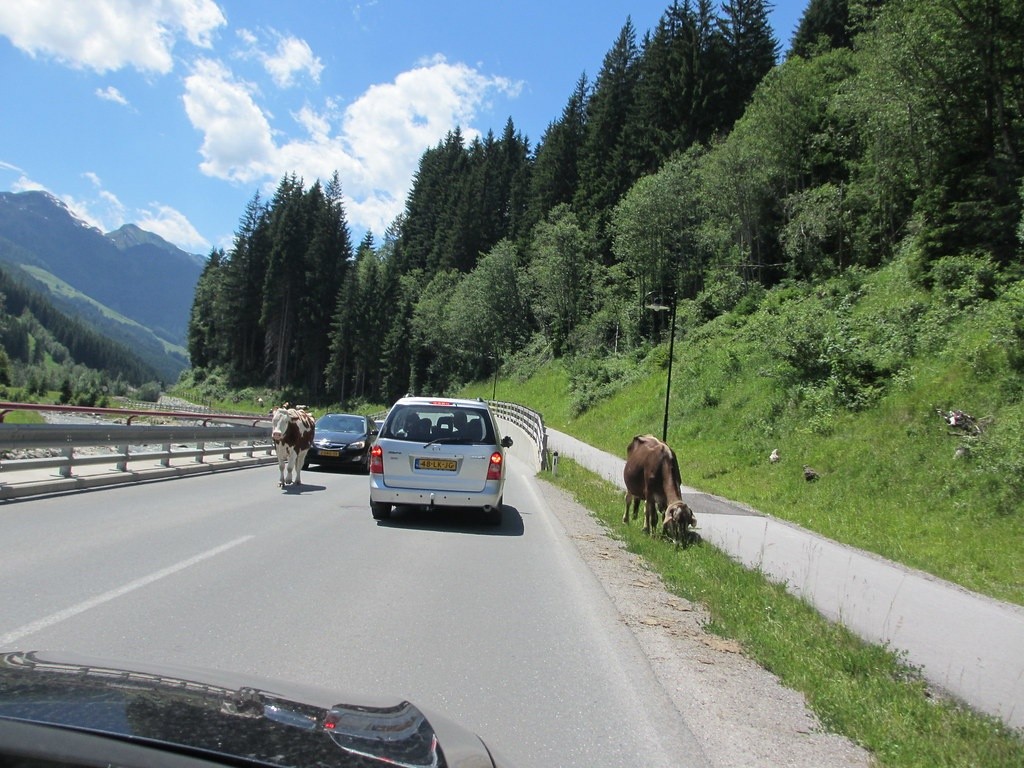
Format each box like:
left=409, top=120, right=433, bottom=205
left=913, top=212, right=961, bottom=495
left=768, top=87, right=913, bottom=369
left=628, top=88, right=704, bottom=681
left=622, top=434, right=698, bottom=549
left=270, top=401, right=315, bottom=488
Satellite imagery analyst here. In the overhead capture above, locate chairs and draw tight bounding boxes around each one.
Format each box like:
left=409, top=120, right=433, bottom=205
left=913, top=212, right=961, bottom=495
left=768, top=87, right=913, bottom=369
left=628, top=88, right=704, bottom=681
left=437, top=417, right=453, bottom=436
left=467, top=419, right=482, bottom=441
left=410, top=418, right=432, bottom=438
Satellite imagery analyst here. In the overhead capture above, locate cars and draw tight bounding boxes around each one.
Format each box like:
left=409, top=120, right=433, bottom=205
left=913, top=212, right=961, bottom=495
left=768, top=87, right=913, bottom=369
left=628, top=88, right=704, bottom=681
left=300, top=413, right=379, bottom=475
left=371, top=420, right=397, bottom=438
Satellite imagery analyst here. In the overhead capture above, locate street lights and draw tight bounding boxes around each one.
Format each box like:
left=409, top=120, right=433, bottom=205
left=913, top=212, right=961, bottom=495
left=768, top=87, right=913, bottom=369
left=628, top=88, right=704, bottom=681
left=487, top=351, right=498, bottom=401
left=640, top=290, right=678, bottom=444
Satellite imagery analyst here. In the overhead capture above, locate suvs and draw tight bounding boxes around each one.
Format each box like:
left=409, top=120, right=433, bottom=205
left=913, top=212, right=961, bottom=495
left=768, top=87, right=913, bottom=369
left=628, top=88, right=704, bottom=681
left=369, top=393, right=513, bottom=526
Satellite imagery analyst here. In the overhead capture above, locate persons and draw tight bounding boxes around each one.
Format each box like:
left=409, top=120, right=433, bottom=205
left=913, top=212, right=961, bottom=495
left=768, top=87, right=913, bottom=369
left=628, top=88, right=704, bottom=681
left=454, top=411, right=467, bottom=430
left=399, top=414, right=420, bottom=436
